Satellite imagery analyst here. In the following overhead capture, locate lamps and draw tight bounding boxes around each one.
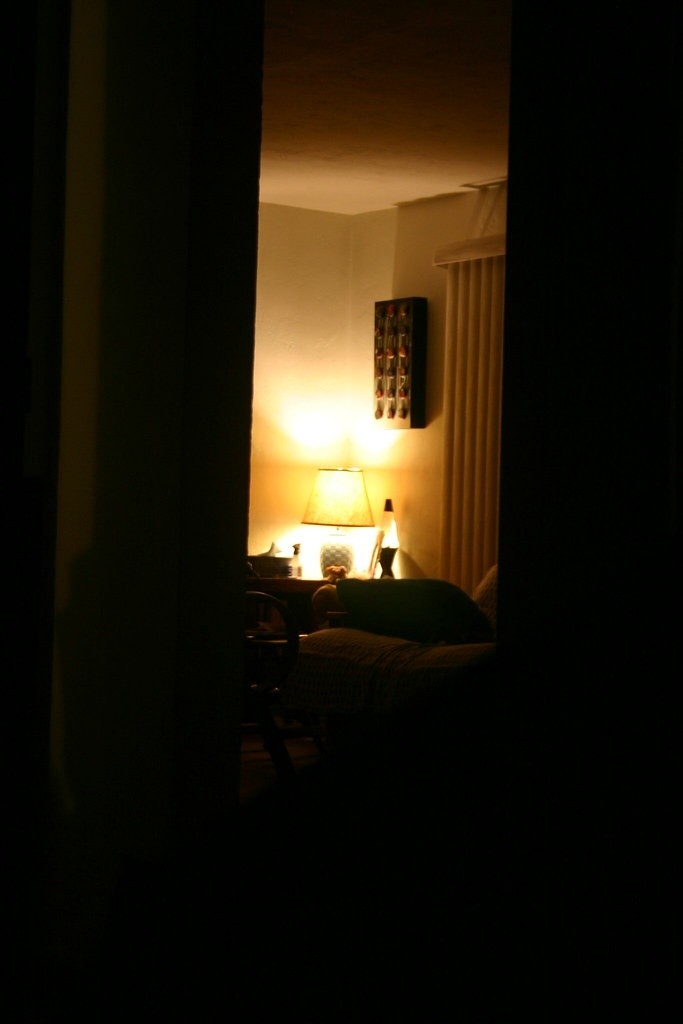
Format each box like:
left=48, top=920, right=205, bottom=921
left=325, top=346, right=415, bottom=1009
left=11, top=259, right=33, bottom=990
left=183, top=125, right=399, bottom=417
left=303, top=467, right=374, bottom=579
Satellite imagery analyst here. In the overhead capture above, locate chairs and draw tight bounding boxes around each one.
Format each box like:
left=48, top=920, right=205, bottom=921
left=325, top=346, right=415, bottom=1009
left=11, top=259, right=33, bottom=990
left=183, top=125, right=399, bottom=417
left=247, top=590, right=291, bottom=691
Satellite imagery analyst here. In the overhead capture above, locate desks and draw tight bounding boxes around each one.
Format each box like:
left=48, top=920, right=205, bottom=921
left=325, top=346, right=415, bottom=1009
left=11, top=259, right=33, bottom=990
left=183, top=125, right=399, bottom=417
left=247, top=576, right=363, bottom=637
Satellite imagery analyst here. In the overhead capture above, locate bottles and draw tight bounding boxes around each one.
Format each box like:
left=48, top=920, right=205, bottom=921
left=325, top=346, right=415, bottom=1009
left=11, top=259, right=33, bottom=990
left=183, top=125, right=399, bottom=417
left=288, top=542, right=302, bottom=578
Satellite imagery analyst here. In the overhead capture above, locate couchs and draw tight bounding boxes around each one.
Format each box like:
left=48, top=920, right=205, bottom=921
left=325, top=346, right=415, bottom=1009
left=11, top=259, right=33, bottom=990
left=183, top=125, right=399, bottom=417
left=289, top=565, right=497, bottom=754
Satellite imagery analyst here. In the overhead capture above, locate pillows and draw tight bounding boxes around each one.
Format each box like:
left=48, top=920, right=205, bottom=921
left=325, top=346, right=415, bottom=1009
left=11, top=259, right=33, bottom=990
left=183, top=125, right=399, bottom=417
left=333, top=575, right=495, bottom=649
